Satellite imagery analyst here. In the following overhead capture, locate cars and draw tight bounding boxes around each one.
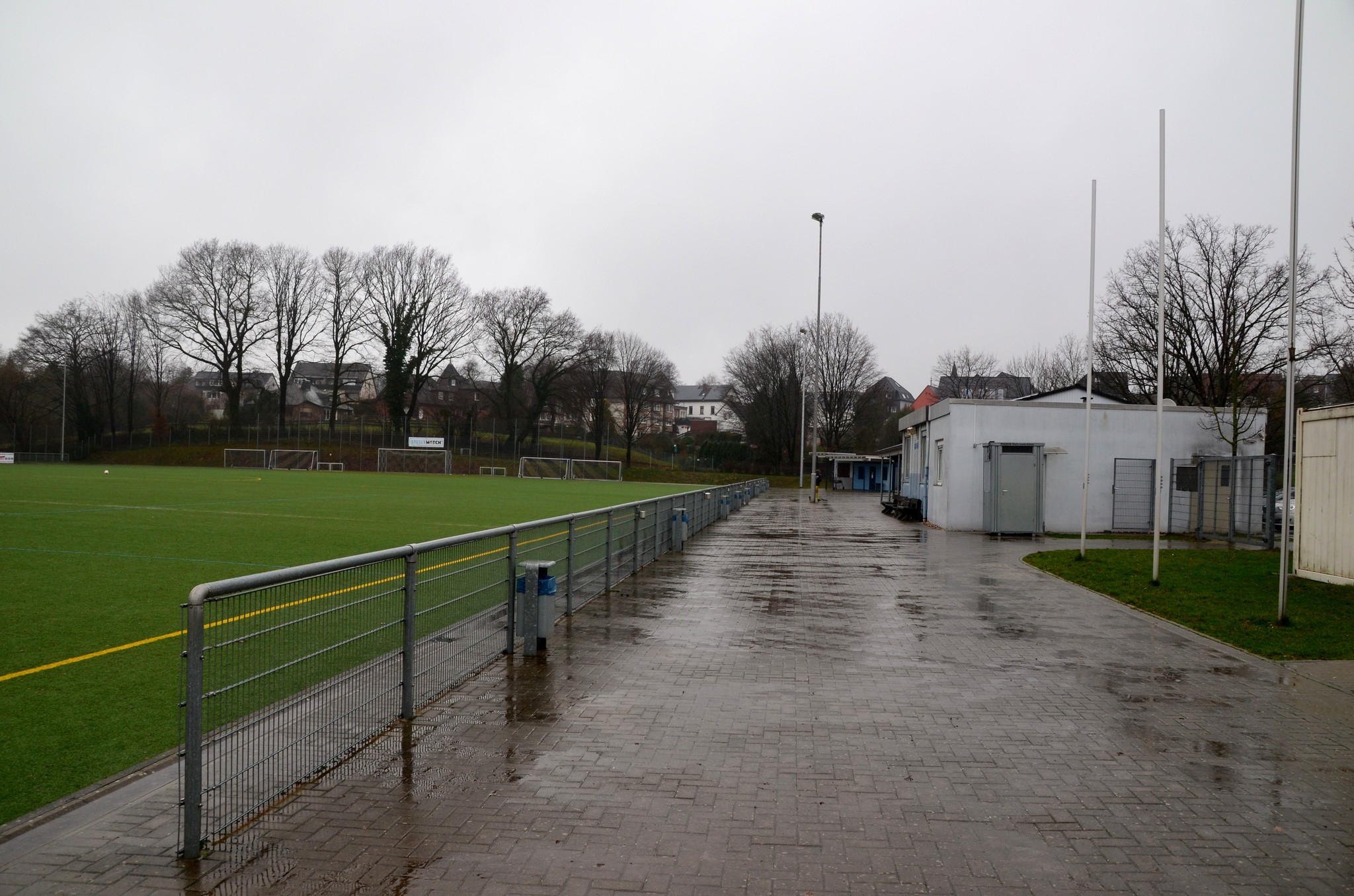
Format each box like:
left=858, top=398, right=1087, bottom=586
left=1262, top=486, right=1295, bottom=531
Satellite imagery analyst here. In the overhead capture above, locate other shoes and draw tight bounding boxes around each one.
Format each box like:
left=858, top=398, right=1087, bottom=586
left=809, top=496, right=810, bottom=500
left=818, top=499, right=821, bottom=501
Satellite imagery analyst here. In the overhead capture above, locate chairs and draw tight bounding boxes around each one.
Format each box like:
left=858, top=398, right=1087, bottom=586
left=881, top=495, right=923, bottom=521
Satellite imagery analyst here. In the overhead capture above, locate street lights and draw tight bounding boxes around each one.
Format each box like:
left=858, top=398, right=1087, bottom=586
left=799, top=328, right=807, bottom=488
left=811, top=210, right=826, bottom=503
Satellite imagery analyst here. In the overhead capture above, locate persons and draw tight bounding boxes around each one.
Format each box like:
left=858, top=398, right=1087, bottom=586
left=809, top=469, right=822, bottom=501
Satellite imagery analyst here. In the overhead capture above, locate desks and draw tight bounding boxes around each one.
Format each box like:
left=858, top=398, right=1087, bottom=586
left=871, top=483, right=884, bottom=491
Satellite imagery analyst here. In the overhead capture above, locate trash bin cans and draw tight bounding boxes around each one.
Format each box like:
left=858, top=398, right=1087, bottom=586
left=672, top=508, right=688, bottom=552
left=876, top=483, right=883, bottom=492
left=766, top=481, right=769, bottom=489
left=762, top=482, right=765, bottom=493
left=744, top=488, right=751, bottom=505
left=754, top=485, right=760, bottom=497
left=516, top=560, right=557, bottom=657
left=734, top=491, right=742, bottom=511
left=720, top=496, right=730, bottom=520
